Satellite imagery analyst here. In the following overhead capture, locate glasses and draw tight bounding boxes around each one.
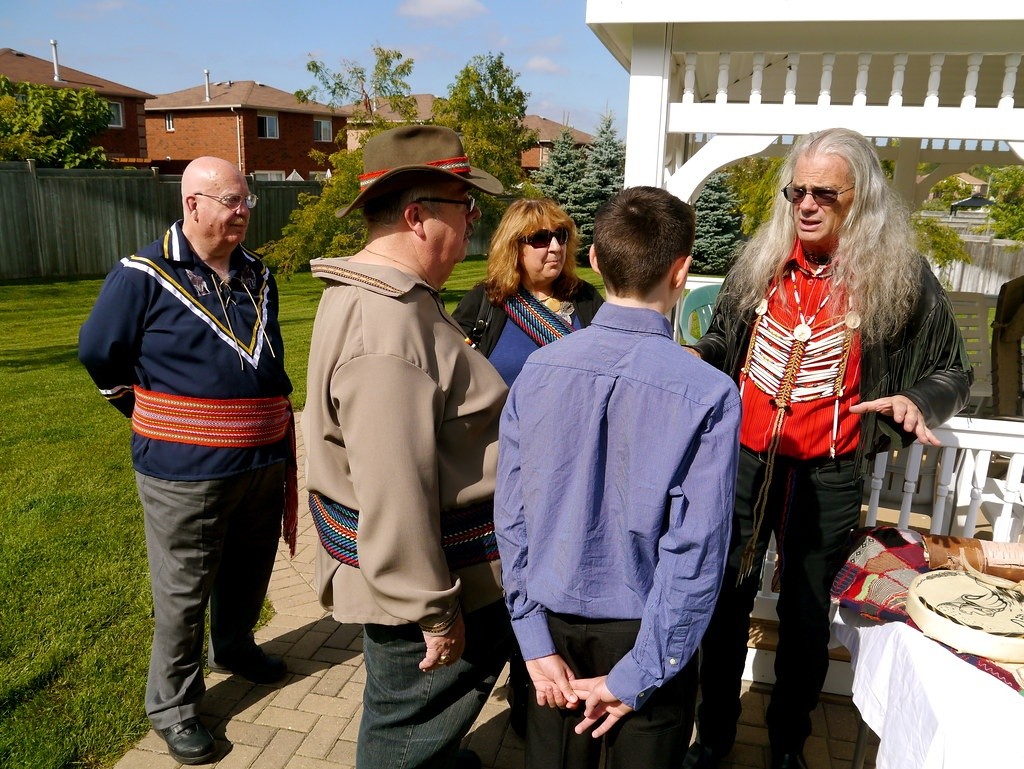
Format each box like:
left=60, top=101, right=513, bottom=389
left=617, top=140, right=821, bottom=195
left=519, top=227, right=569, bottom=248
left=194, top=192, right=259, bottom=212
left=402, top=194, right=475, bottom=218
left=781, top=180, right=854, bottom=206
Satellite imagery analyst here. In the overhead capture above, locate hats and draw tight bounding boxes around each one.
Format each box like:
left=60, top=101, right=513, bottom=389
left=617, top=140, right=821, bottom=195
left=334, top=125, right=504, bottom=218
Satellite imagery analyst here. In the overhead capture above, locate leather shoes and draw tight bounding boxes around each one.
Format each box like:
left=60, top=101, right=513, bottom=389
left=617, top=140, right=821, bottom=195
left=154, top=716, right=218, bottom=764
left=209, top=646, right=287, bottom=685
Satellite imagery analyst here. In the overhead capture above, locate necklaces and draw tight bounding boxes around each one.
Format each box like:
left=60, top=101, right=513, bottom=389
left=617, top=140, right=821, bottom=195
left=794, top=268, right=837, bottom=278
left=539, top=292, right=560, bottom=312
left=364, top=249, right=426, bottom=281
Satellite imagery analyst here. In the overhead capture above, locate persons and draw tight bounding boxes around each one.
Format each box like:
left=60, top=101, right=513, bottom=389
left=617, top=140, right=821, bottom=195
left=493, top=186, right=741, bottom=769
left=79, top=156, right=298, bottom=764
left=680, top=128, right=974, bottom=769
left=302, top=126, right=510, bottom=769
left=452, top=197, right=605, bottom=390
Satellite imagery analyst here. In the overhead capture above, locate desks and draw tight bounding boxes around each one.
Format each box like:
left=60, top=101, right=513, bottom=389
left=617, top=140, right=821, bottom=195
left=831, top=605, right=1024, bottom=769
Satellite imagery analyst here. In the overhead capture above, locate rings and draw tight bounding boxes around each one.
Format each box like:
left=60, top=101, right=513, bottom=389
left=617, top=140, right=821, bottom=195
left=437, top=655, right=449, bottom=664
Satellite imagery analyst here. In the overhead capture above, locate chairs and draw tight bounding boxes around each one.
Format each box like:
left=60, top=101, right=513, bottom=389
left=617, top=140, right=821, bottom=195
left=679, top=284, right=723, bottom=346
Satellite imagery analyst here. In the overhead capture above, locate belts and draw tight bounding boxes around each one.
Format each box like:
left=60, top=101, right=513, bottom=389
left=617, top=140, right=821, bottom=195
left=739, top=444, right=855, bottom=470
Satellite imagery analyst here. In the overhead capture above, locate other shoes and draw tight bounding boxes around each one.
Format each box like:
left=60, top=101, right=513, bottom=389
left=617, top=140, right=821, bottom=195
left=456, top=748, right=482, bottom=769
left=770, top=749, right=808, bottom=769
left=681, top=741, right=732, bottom=769
